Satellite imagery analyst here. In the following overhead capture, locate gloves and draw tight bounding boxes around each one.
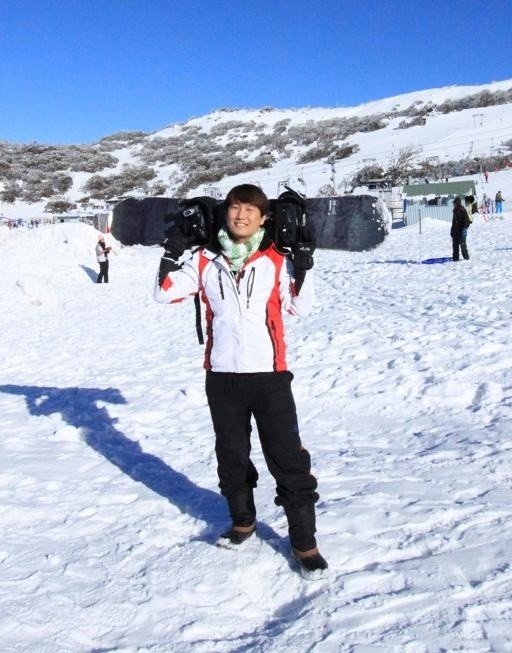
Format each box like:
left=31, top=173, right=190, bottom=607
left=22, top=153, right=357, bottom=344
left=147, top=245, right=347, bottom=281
left=291, top=223, right=317, bottom=270
left=159, top=221, right=195, bottom=257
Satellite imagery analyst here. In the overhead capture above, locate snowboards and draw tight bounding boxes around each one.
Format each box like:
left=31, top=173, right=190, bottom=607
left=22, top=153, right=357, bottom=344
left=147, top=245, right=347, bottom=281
left=109, top=194, right=392, bottom=253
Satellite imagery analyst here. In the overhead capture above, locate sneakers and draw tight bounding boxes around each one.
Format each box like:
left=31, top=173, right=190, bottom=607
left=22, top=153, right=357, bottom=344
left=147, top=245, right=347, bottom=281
left=289, top=545, right=330, bottom=582
left=212, top=522, right=257, bottom=552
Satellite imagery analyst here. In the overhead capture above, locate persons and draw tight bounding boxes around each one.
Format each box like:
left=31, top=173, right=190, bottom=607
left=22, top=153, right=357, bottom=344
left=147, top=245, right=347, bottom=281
left=155, top=184, right=330, bottom=581
left=427, top=164, right=505, bottom=261
left=7, top=217, right=52, bottom=231
left=96, top=234, right=112, bottom=283
